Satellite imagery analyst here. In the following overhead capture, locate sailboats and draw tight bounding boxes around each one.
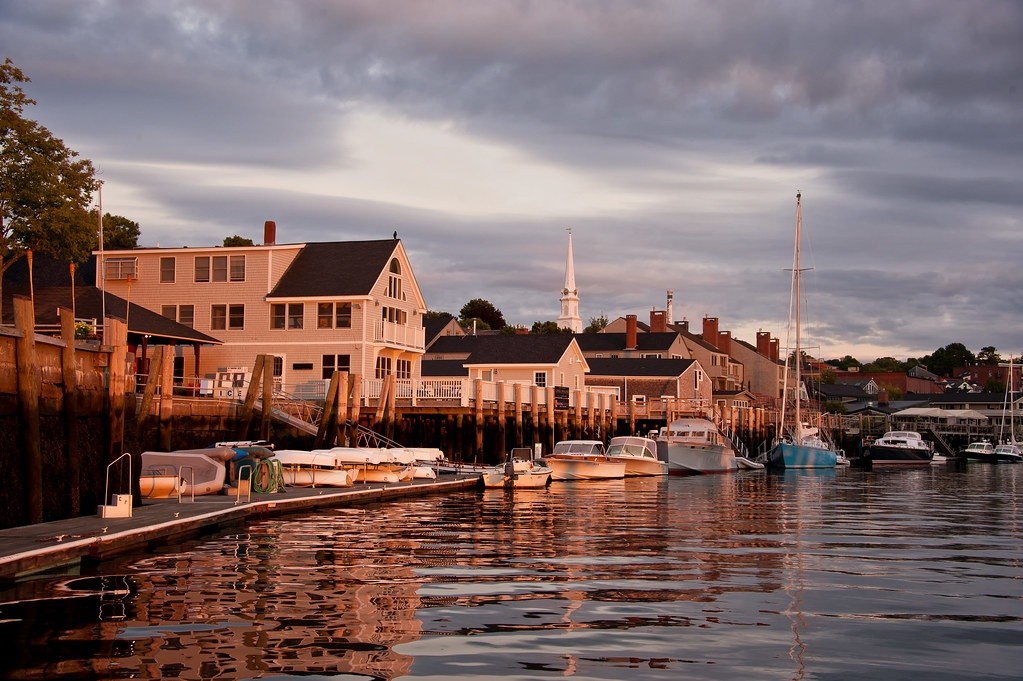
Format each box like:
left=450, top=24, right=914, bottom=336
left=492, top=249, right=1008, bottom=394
left=985, top=352, right=1023, bottom=463
left=734, top=188, right=852, bottom=471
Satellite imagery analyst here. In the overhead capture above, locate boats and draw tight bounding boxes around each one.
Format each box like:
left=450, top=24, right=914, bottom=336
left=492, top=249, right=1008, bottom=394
left=869, top=429, right=935, bottom=464
left=958, top=439, right=997, bottom=461
left=138, top=445, right=460, bottom=499
left=540, top=439, right=627, bottom=482
left=481, top=448, right=554, bottom=489
left=652, top=405, right=739, bottom=477
left=605, top=430, right=669, bottom=478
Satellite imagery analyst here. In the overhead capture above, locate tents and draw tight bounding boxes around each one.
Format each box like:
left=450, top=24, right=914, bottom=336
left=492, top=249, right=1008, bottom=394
left=892, top=407, right=950, bottom=418
left=944, top=408, right=989, bottom=420
